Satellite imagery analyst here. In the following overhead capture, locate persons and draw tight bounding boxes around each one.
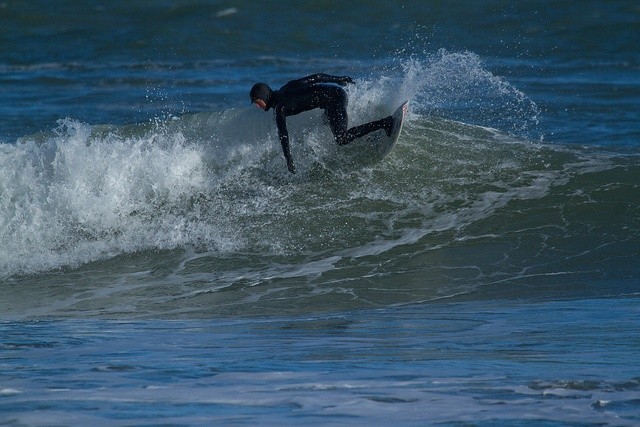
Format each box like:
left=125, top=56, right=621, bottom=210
left=249, top=73, right=394, bottom=174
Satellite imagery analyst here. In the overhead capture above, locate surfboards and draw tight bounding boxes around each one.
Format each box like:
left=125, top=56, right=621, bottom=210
left=358, top=100, right=407, bottom=169
